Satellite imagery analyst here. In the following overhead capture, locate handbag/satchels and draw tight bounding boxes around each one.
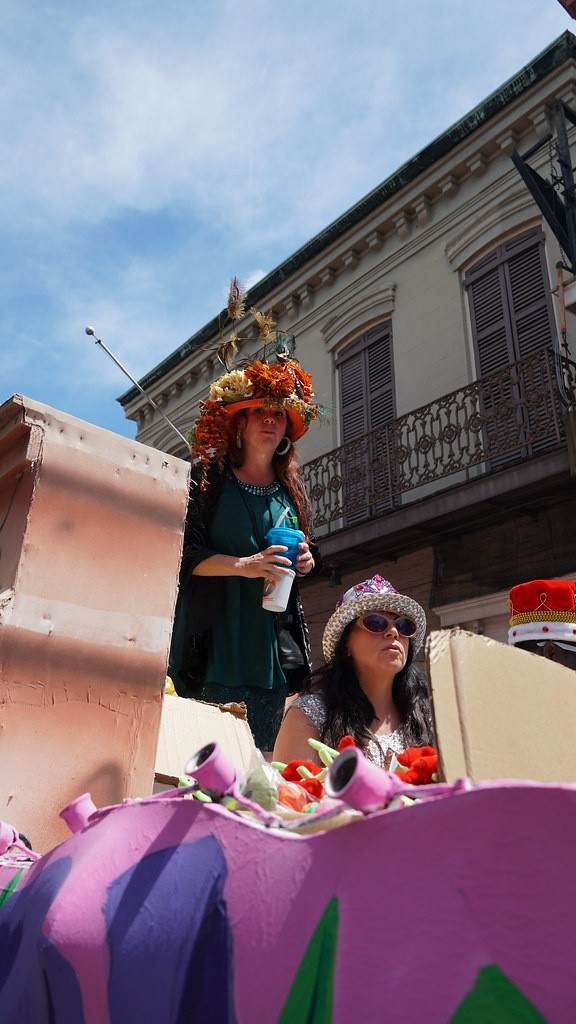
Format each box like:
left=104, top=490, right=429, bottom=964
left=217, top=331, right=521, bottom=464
left=274, top=580, right=313, bottom=693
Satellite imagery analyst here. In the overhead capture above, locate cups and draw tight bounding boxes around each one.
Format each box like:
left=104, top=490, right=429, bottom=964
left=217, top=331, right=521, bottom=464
left=262, top=567, right=297, bottom=612
left=264, top=527, right=305, bottom=570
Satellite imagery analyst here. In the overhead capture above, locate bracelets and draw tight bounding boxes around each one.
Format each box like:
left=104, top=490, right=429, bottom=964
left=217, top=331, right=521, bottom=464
left=296, top=563, right=313, bottom=575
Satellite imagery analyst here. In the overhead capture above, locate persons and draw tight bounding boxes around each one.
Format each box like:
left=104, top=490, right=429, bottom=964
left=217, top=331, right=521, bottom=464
left=508, top=579, right=576, bottom=670
left=272, top=573, right=434, bottom=773
left=166, top=359, right=322, bottom=763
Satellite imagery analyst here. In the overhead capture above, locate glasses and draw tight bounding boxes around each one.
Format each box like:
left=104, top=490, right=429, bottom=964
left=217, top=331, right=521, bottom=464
left=350, top=612, right=418, bottom=638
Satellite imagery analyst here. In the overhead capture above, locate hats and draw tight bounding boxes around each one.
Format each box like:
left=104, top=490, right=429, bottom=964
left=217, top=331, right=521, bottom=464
left=508, top=580, right=576, bottom=645
left=190, top=278, right=334, bottom=492
left=322, top=574, right=427, bottom=670
